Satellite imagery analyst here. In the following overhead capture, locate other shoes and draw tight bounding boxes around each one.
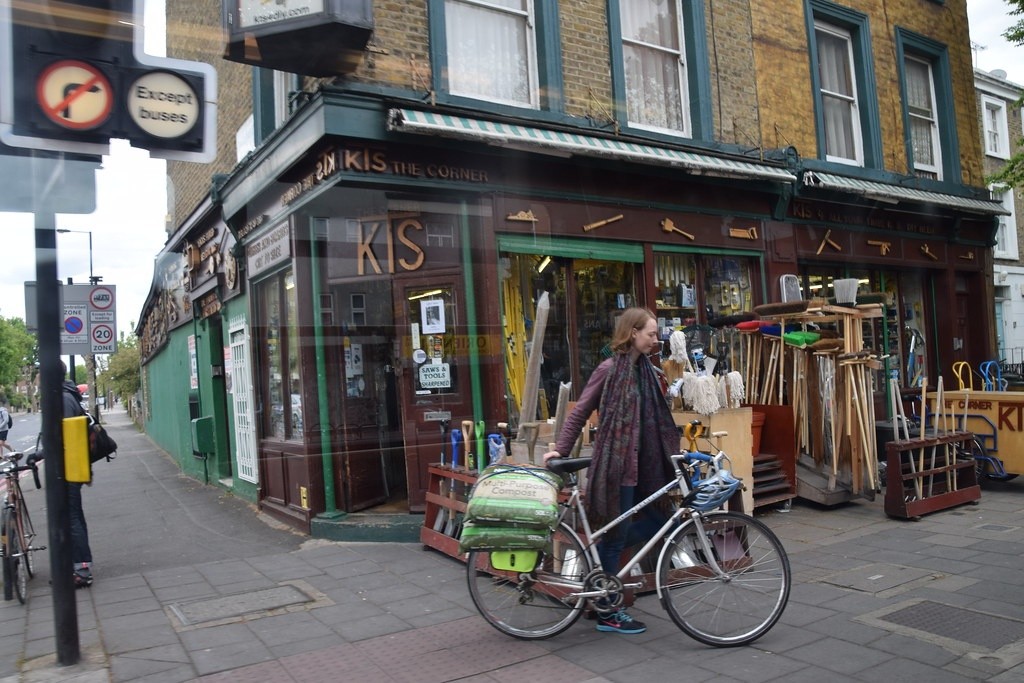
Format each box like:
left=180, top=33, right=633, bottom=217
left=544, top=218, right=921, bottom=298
left=49, top=566, right=93, bottom=588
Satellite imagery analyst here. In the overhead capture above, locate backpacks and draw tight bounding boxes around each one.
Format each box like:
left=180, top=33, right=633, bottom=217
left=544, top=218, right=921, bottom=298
left=1, top=411, right=13, bottom=429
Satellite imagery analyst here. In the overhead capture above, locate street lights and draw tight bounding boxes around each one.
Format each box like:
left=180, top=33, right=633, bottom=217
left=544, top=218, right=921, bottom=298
left=55, top=227, right=100, bottom=421
left=101, top=376, right=115, bottom=410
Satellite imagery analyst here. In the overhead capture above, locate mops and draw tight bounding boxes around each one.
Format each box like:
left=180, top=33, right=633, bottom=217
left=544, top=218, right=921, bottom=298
left=668, top=330, right=745, bottom=416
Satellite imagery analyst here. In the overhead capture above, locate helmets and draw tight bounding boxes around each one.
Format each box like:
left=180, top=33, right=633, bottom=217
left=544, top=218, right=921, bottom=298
left=679, top=470, right=742, bottom=509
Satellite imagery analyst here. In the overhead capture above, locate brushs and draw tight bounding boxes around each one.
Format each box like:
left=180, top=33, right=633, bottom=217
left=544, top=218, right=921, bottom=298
left=708, top=301, right=841, bottom=480
left=837, top=348, right=878, bottom=498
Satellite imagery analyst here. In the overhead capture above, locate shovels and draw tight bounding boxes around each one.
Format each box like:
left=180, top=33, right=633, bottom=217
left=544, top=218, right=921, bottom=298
left=431, top=418, right=483, bottom=545
left=490, top=421, right=751, bottom=584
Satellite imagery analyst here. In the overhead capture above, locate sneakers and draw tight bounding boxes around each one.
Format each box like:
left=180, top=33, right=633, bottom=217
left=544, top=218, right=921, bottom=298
left=597, top=611, right=647, bottom=632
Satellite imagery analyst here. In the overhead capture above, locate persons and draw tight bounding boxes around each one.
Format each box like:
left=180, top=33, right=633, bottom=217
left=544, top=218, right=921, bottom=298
left=0, top=406, right=16, bottom=460
left=27, top=360, right=94, bottom=587
left=544, top=310, right=681, bottom=634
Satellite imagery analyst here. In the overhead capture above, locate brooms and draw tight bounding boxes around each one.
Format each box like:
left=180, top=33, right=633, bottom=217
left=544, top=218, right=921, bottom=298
left=707, top=278, right=885, bottom=489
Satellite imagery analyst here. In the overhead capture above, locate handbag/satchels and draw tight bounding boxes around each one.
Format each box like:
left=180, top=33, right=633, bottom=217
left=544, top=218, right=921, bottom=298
left=87, top=414, right=117, bottom=462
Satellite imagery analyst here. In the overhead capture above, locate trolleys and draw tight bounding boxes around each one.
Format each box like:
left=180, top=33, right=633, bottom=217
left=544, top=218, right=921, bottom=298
left=903, top=360, right=1009, bottom=490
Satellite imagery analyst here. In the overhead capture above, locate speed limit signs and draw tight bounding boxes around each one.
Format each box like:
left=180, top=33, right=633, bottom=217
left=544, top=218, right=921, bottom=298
left=89, top=322, right=115, bottom=353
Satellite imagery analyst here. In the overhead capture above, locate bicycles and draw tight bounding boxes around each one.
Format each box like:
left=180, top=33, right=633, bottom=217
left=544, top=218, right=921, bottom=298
left=0, top=451, right=48, bottom=605
left=466, top=430, right=793, bottom=648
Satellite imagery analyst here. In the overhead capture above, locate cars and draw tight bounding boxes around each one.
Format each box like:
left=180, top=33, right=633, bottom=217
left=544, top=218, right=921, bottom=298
left=80, top=394, right=90, bottom=412
